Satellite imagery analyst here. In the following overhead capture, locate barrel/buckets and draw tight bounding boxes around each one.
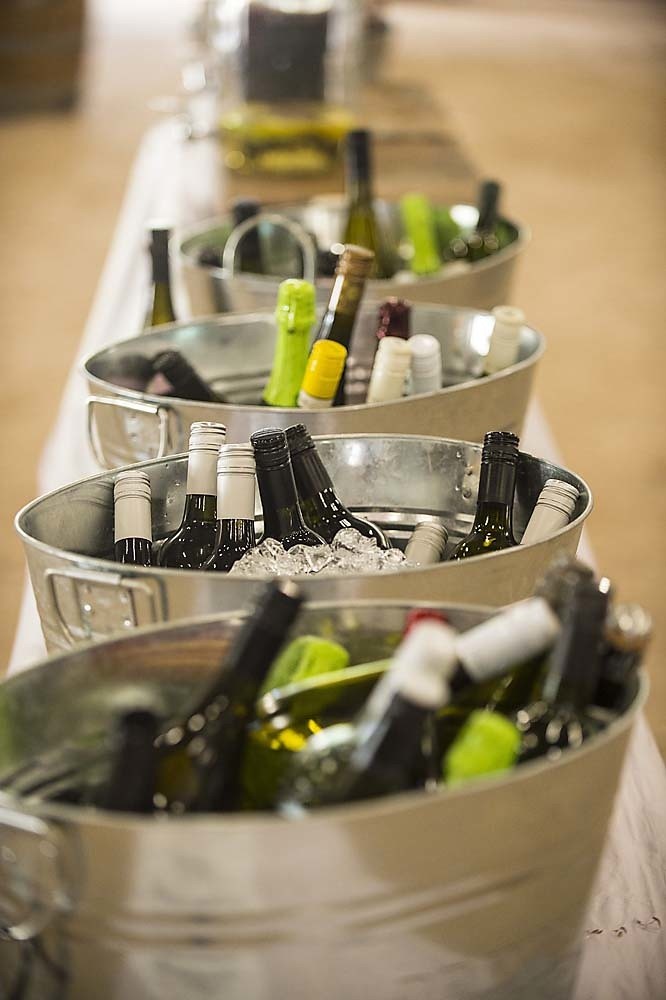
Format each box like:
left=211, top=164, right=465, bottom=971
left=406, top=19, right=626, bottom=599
left=81, top=301, right=545, bottom=470
left=171, top=191, right=527, bottom=307
left=8, top=432, right=593, bottom=653
left=0, top=600, right=651, bottom=1000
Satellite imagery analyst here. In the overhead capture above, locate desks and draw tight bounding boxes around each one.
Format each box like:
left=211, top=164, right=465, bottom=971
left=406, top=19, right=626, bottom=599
left=0, top=116, right=666, bottom=1000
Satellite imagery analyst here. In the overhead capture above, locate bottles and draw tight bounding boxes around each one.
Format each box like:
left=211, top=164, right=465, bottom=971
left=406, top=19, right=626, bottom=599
left=56, top=128, right=658, bottom=826
